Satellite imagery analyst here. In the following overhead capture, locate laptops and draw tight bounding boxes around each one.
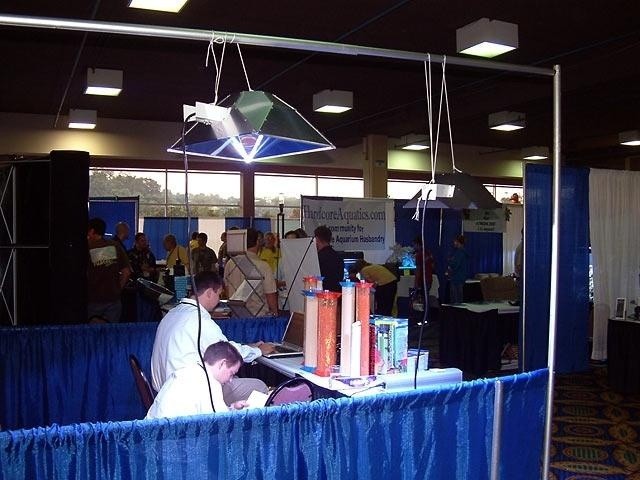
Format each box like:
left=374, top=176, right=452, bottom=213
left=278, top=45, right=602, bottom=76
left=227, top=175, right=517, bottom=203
left=263, top=311, right=305, bottom=359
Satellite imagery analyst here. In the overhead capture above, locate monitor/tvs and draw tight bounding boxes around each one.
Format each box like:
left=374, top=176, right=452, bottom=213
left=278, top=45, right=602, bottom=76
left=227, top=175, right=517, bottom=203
left=342, top=252, right=365, bottom=282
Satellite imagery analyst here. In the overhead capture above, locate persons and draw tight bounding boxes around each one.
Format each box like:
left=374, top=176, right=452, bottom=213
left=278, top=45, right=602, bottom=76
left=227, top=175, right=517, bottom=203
left=315, top=226, right=344, bottom=292
left=192, top=233, right=217, bottom=272
left=127, top=233, right=155, bottom=288
left=264, top=233, right=282, bottom=266
left=190, top=232, right=200, bottom=255
left=446, top=233, right=475, bottom=299
left=164, top=234, right=189, bottom=293
left=88, top=218, right=131, bottom=324
left=356, top=260, right=398, bottom=316
left=256, top=232, right=276, bottom=278
left=510, top=193, right=518, bottom=203
left=414, top=237, right=433, bottom=327
left=151, top=272, right=268, bottom=407
left=112, top=221, right=133, bottom=321
left=219, top=233, right=229, bottom=278
left=224, top=228, right=277, bottom=318
left=147, top=341, right=250, bottom=419
left=516, top=228, right=524, bottom=276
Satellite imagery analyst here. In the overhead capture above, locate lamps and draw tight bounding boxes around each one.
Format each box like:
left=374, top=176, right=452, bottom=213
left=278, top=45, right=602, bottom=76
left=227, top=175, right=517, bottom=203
left=400, top=134, right=430, bottom=152
left=170, top=45, right=337, bottom=166
left=66, top=108, right=98, bottom=130
left=402, top=50, right=508, bottom=216
left=617, top=130, right=640, bottom=147
left=127, top=0, right=189, bottom=14
left=456, top=18, right=518, bottom=59
left=488, top=111, right=525, bottom=133
left=82, top=67, right=123, bottom=98
left=312, top=89, right=353, bottom=115
left=520, top=146, right=550, bottom=161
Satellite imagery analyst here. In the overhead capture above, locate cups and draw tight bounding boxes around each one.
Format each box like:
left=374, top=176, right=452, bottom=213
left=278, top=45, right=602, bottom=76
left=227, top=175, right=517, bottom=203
left=329, top=364, right=344, bottom=391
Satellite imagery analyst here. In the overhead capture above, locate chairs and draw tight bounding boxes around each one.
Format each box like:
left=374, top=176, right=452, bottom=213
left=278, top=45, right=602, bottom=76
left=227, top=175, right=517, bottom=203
left=265, top=377, right=315, bottom=406
left=127, top=352, right=155, bottom=412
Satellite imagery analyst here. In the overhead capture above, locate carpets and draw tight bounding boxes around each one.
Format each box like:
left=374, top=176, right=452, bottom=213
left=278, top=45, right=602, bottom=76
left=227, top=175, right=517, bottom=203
left=409, top=341, right=640, bottom=479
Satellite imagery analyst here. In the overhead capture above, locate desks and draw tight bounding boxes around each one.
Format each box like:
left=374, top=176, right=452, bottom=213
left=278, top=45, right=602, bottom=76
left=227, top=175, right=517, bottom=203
left=438, top=299, right=521, bottom=378
left=257, top=341, right=463, bottom=400
left=606, top=317, right=640, bottom=396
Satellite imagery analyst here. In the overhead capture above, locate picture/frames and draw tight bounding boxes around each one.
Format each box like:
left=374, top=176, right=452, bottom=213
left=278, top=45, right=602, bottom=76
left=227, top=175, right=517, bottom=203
left=614, top=297, right=626, bottom=320
